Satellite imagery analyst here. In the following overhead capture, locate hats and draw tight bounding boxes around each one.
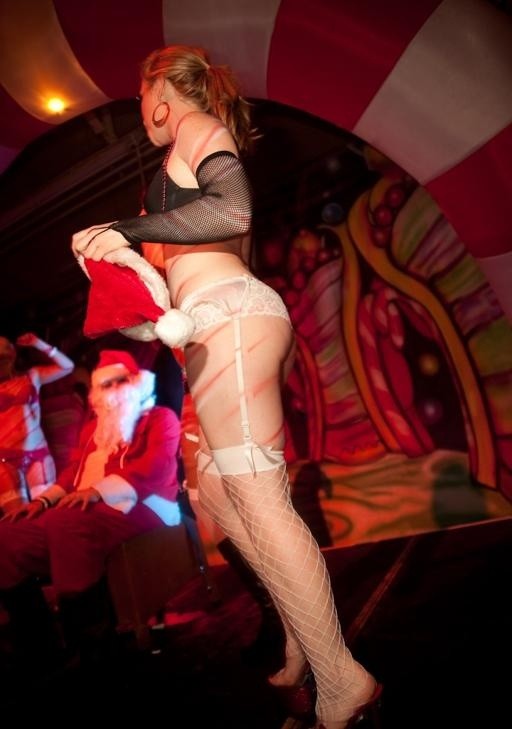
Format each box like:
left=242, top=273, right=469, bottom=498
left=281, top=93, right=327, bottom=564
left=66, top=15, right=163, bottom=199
left=92, top=349, right=142, bottom=392
left=77, top=246, right=196, bottom=350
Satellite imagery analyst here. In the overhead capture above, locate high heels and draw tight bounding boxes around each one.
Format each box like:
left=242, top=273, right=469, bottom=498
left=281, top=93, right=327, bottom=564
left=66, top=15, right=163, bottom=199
left=312, top=681, right=385, bottom=729
left=267, top=662, right=313, bottom=690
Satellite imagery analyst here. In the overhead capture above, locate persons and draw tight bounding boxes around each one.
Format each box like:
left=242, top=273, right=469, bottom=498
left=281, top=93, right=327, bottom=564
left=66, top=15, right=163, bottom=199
left=0, top=333, right=75, bottom=512
left=0, top=348, right=182, bottom=672
left=69, top=42, right=383, bottom=729
